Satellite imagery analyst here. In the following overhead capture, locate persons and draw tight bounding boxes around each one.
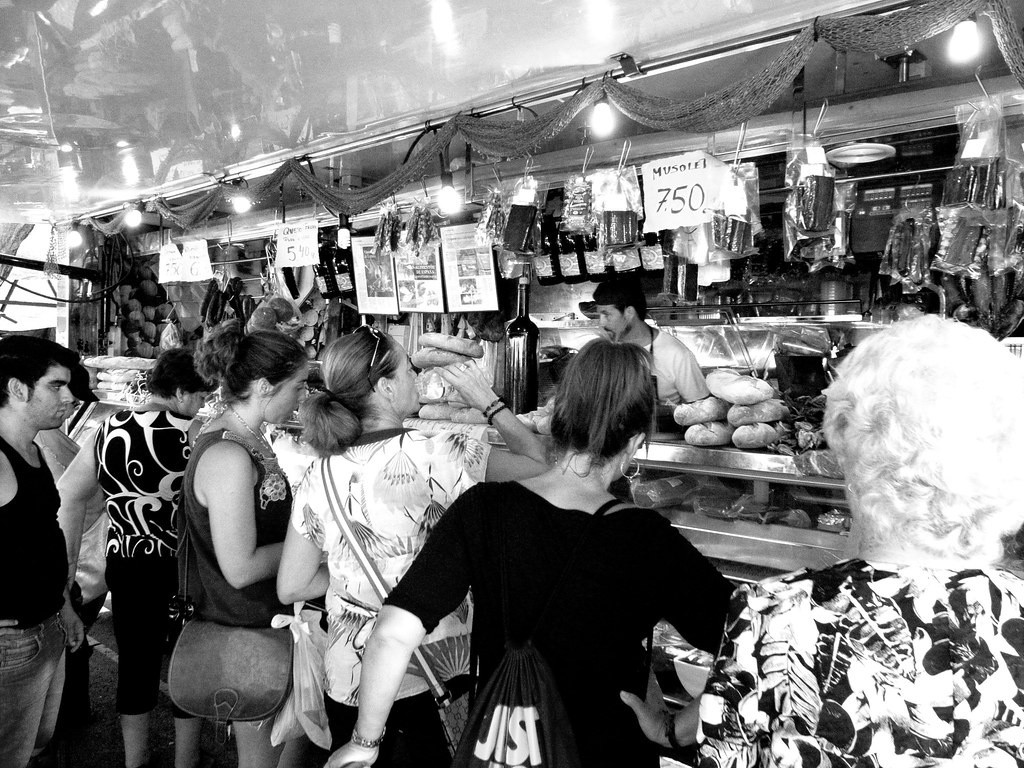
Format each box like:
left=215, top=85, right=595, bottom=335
left=593, top=280, right=710, bottom=418
left=693, top=314, right=1024, bottom=768
left=172, top=327, right=310, bottom=768
left=36, top=366, right=109, bottom=729
left=277, top=324, right=557, bottom=768
left=57, top=348, right=219, bottom=768
left=0, top=335, right=85, bottom=768
left=321, top=338, right=736, bottom=768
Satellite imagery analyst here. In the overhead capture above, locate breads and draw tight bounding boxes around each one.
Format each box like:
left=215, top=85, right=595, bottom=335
left=633, top=476, right=850, bottom=531
left=411, top=333, right=557, bottom=434
left=673, top=368, right=789, bottom=448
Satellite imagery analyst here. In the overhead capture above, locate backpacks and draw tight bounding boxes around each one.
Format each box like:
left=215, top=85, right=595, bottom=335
left=451, top=481, right=654, bottom=768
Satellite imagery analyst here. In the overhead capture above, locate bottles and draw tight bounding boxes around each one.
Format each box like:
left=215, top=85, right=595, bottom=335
left=527, top=202, right=666, bottom=283
left=414, top=367, right=492, bottom=401
left=503, top=278, right=540, bottom=418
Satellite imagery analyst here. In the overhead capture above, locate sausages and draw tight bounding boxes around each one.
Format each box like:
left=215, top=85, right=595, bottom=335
left=199, top=276, right=244, bottom=327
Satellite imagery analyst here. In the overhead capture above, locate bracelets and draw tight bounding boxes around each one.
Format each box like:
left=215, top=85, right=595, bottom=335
left=665, top=714, right=682, bottom=748
left=351, top=722, right=386, bottom=748
left=483, top=398, right=511, bottom=426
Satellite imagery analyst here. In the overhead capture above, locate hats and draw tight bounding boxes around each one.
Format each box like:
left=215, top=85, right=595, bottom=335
left=67, top=364, right=101, bottom=402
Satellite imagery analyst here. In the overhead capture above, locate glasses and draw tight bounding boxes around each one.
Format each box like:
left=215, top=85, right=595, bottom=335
left=353, top=324, right=386, bottom=392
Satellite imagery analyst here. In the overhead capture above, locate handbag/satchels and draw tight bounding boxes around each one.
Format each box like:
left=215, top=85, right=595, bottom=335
left=167, top=618, right=294, bottom=746
left=270, top=599, right=340, bottom=751
left=438, top=683, right=479, bottom=758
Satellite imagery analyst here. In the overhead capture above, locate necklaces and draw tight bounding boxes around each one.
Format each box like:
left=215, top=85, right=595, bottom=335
left=230, top=405, right=273, bottom=454
